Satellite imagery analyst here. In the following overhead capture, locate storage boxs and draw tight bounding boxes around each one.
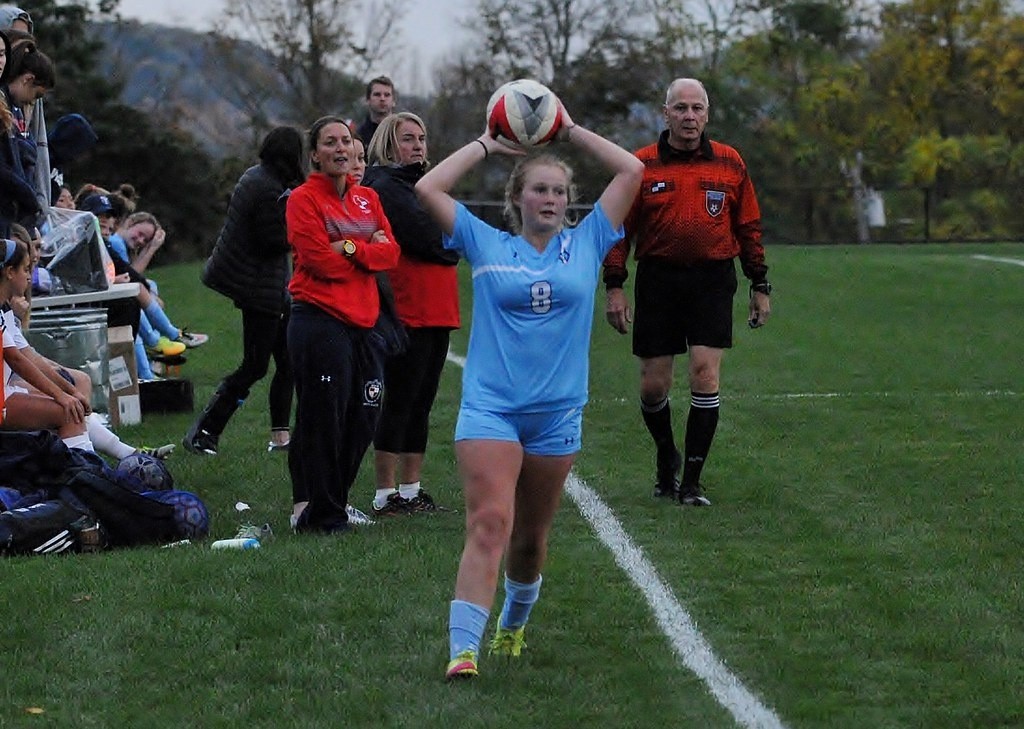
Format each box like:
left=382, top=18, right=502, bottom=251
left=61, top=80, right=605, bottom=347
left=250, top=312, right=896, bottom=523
left=106, top=325, right=142, bottom=426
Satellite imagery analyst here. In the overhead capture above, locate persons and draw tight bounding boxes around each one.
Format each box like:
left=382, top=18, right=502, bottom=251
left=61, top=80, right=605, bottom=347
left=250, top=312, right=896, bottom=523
left=0, top=7, right=208, bottom=461
left=179, top=123, right=292, bottom=457
left=604, top=77, right=770, bottom=506
left=415, top=96, right=646, bottom=680
left=285, top=74, right=461, bottom=535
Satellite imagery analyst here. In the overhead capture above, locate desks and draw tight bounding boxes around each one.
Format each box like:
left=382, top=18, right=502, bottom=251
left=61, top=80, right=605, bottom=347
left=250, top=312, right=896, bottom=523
left=28, top=280, right=140, bottom=333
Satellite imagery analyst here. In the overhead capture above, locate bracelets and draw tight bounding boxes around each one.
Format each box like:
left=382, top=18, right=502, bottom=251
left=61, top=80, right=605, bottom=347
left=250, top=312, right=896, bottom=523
left=559, top=122, right=576, bottom=142
left=474, top=140, right=488, bottom=159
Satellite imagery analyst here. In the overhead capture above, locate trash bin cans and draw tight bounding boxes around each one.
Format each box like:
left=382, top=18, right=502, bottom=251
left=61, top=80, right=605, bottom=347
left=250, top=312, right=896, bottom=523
left=28, top=308, right=111, bottom=429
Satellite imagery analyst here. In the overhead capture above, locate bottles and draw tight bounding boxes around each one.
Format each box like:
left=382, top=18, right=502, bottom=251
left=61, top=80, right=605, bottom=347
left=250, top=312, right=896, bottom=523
left=210, top=538, right=262, bottom=552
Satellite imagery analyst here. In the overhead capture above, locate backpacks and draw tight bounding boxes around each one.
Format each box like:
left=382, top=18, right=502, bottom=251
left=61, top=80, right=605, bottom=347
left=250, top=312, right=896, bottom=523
left=0, top=430, right=176, bottom=560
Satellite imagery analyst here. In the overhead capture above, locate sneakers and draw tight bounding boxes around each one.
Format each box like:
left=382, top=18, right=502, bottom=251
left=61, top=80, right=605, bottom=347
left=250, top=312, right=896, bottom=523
left=146, top=336, right=185, bottom=356
left=487, top=615, right=527, bottom=657
left=371, top=492, right=419, bottom=520
left=444, top=650, right=478, bottom=679
left=132, top=443, right=176, bottom=460
left=410, top=490, right=458, bottom=515
left=174, top=327, right=209, bottom=348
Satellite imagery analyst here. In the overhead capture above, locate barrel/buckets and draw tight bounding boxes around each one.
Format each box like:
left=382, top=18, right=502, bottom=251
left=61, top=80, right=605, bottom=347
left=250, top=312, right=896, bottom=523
left=26, top=308, right=114, bottom=436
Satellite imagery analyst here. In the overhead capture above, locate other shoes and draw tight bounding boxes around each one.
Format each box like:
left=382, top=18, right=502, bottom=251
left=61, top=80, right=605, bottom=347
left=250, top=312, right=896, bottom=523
left=654, top=472, right=679, bottom=501
left=346, top=505, right=370, bottom=519
left=290, top=514, right=306, bottom=527
left=267, top=439, right=291, bottom=453
left=182, top=437, right=217, bottom=457
left=145, top=346, right=186, bottom=365
left=679, top=483, right=711, bottom=507
left=335, top=513, right=376, bottom=536
left=233, top=521, right=274, bottom=547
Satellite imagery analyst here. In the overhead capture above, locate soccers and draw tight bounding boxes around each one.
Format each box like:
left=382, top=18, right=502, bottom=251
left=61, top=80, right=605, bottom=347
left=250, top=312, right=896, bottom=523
left=486, top=78, right=562, bottom=151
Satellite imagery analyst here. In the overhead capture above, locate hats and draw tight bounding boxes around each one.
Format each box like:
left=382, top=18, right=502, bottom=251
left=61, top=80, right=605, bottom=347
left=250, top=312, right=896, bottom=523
left=79, top=194, right=114, bottom=216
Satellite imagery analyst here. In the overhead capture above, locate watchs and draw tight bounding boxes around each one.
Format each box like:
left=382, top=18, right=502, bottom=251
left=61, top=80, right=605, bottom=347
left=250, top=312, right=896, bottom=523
left=343, top=240, right=356, bottom=257
left=749, top=283, right=773, bottom=299
